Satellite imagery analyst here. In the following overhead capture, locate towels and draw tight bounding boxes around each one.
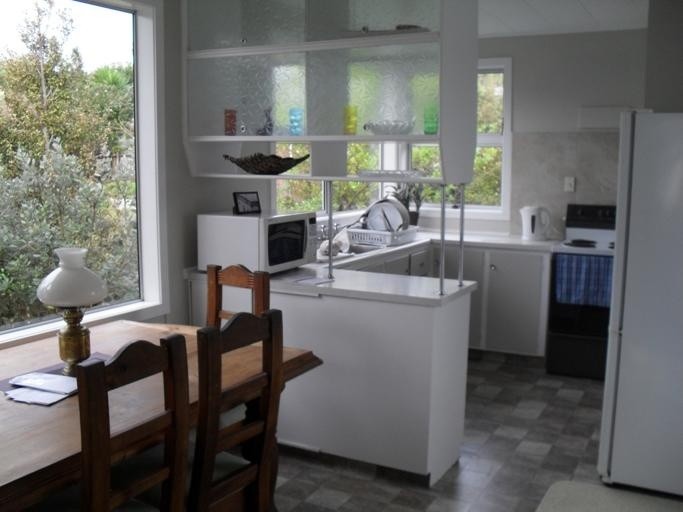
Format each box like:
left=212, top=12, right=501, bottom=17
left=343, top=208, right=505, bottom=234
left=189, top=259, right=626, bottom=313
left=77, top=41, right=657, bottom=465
left=557, top=255, right=613, bottom=307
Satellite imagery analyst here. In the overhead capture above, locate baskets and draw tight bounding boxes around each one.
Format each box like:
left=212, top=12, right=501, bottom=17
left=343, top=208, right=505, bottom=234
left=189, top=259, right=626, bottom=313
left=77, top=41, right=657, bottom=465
left=347, top=225, right=420, bottom=247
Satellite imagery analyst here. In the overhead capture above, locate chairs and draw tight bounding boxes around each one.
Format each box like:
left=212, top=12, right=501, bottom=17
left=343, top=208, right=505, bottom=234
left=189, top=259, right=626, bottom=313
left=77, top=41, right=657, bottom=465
left=189, top=264, right=269, bottom=443
left=24, top=336, right=189, bottom=512
left=111, top=310, right=282, bottom=512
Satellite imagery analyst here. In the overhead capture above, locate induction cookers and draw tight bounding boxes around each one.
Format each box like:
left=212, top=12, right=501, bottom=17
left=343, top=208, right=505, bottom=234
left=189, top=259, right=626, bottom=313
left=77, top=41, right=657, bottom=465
left=552, top=229, right=615, bottom=258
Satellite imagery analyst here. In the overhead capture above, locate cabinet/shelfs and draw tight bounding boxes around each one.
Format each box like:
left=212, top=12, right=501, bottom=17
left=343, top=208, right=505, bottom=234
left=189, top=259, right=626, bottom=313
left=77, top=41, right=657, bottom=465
left=477, top=0, right=650, bottom=37
left=483, top=249, right=549, bottom=357
left=433, top=247, right=486, bottom=350
left=358, top=251, right=433, bottom=277
left=182, top=0, right=477, bottom=183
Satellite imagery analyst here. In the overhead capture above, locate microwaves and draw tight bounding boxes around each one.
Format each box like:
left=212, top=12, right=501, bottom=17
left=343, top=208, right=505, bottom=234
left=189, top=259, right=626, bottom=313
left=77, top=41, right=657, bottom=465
left=196, top=209, right=318, bottom=276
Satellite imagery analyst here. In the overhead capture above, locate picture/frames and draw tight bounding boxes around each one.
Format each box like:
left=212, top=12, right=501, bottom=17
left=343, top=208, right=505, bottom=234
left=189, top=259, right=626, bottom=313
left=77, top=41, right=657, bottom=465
left=233, top=192, right=261, bottom=214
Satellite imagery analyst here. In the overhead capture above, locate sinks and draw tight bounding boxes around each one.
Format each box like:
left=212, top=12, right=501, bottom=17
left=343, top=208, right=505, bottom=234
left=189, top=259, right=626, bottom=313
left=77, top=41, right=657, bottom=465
left=343, top=240, right=379, bottom=255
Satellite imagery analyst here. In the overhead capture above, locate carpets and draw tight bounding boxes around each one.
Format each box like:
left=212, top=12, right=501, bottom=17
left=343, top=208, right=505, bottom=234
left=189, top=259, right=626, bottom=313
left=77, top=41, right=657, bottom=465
left=536, top=481, right=683, bottom=512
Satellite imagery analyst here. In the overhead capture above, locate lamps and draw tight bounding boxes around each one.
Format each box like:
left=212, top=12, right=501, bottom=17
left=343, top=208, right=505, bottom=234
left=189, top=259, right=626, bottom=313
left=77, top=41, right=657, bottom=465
left=37, top=247, right=107, bottom=375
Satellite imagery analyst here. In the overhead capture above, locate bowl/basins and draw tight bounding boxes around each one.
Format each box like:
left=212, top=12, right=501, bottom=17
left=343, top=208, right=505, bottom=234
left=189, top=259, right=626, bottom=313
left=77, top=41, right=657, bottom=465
left=365, top=120, right=414, bottom=135
left=221, top=151, right=310, bottom=174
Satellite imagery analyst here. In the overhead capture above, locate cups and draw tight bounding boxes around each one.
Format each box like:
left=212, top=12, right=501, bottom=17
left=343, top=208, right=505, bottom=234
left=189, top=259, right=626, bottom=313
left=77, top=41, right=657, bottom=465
left=343, top=104, right=357, bottom=135
left=423, top=107, right=437, bottom=135
left=288, top=107, right=304, bottom=135
left=222, top=108, right=236, bottom=135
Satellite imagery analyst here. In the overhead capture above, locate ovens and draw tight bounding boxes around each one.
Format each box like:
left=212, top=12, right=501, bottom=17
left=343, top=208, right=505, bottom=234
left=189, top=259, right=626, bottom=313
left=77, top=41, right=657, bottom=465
left=544, top=253, right=613, bottom=380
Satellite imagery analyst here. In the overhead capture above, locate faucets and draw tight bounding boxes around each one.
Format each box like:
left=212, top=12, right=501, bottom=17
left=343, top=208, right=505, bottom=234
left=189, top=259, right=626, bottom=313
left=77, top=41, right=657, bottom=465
left=334, top=214, right=368, bottom=238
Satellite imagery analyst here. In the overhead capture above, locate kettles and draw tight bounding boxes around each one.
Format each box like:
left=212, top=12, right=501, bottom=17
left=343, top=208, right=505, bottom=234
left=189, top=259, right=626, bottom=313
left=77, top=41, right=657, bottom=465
left=518, top=205, right=551, bottom=241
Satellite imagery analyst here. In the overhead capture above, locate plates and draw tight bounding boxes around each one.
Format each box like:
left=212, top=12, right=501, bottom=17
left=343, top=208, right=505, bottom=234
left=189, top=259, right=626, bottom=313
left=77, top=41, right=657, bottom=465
left=366, top=199, right=410, bottom=232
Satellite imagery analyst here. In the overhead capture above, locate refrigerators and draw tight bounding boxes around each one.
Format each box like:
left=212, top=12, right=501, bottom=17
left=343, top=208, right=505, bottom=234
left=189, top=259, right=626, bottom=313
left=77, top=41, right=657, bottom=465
left=593, top=105, right=682, bottom=499
